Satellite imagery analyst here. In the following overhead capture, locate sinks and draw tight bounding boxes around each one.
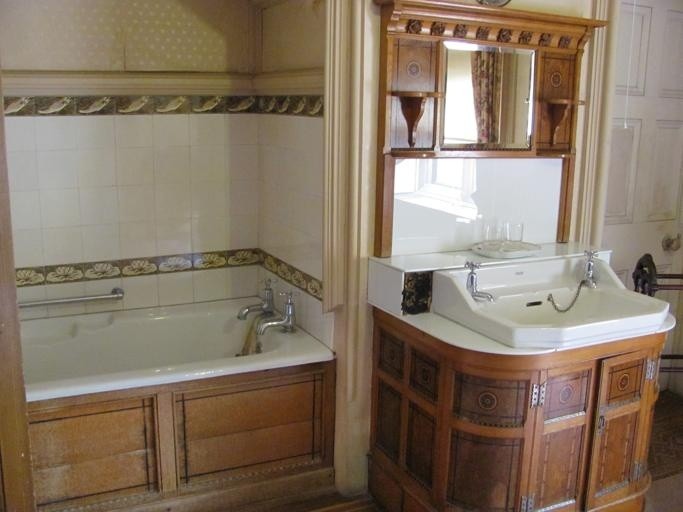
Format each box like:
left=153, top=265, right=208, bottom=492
left=431, top=255, right=669, bottom=350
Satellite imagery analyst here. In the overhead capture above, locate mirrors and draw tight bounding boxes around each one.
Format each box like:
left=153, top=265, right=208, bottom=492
left=442, top=43, right=537, bottom=151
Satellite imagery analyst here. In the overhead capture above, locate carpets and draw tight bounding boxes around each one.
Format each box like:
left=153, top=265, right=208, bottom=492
left=647, top=388, right=682, bottom=484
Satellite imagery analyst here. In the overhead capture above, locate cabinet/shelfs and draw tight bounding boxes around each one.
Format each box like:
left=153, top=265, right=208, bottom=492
left=536, top=50, right=570, bottom=144
left=392, top=37, right=437, bottom=151
left=526, top=346, right=662, bottom=512
left=368, top=319, right=440, bottom=494
left=431, top=363, right=532, bottom=512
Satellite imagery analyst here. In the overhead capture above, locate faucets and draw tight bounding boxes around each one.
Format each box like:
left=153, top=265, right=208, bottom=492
left=583, top=250, right=598, bottom=288
left=256, top=290, right=302, bottom=335
left=464, top=261, right=495, bottom=303
left=238, top=278, right=278, bottom=321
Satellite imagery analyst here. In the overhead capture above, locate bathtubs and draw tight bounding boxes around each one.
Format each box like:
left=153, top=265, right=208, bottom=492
left=19, top=297, right=335, bottom=403
